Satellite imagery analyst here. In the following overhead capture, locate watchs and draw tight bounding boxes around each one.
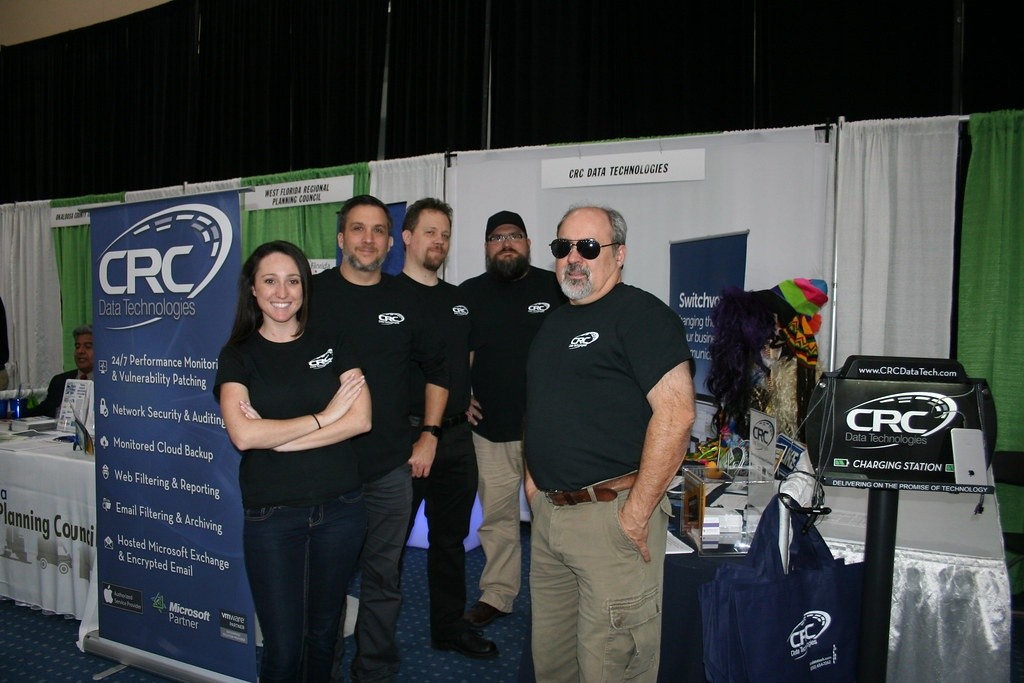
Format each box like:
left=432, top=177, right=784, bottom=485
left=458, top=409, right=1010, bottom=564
left=420, top=425, right=442, bottom=438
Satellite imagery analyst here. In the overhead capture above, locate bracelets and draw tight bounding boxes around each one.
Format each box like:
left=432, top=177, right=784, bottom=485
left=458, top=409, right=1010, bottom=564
left=311, top=413, right=321, bottom=429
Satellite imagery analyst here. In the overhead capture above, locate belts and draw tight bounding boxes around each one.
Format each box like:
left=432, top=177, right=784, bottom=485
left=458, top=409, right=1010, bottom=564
left=442, top=412, right=468, bottom=429
left=546, top=471, right=640, bottom=506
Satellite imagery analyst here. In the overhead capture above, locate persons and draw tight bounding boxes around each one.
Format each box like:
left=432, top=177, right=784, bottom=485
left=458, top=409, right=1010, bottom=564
left=212, top=239, right=372, bottom=683
left=21, top=325, right=94, bottom=420
left=522, top=206, right=697, bottom=683
left=394, top=197, right=499, bottom=660
left=459, top=210, right=570, bottom=627
left=0, top=297, right=9, bottom=391
left=312, top=195, right=450, bottom=683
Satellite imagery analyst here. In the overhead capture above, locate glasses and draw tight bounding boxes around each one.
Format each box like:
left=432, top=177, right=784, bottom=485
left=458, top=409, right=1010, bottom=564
left=487, top=232, right=524, bottom=244
left=549, top=239, right=617, bottom=259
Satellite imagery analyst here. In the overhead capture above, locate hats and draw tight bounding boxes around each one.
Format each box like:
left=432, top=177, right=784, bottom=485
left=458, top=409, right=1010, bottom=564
left=485, top=211, right=529, bottom=238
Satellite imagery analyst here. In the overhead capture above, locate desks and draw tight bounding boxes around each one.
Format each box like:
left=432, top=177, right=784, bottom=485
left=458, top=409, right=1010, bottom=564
left=658, top=451, right=1011, bottom=683
left=0, top=419, right=98, bottom=652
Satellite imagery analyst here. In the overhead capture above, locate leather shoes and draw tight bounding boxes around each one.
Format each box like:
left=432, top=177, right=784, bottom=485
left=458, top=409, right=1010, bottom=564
left=465, top=600, right=509, bottom=626
left=431, top=627, right=498, bottom=660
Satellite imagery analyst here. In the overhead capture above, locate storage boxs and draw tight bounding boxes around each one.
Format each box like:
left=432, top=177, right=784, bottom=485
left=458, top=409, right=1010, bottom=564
left=681, top=463, right=775, bottom=558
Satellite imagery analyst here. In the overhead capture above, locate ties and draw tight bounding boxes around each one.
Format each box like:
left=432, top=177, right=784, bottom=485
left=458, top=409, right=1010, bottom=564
left=80, top=374, right=87, bottom=379
